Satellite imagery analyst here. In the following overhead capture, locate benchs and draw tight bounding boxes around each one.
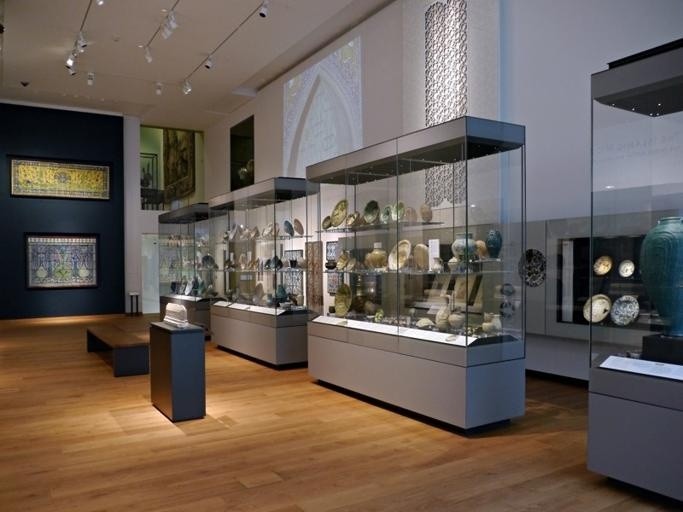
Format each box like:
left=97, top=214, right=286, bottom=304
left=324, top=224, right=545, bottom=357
left=85, top=315, right=149, bottom=378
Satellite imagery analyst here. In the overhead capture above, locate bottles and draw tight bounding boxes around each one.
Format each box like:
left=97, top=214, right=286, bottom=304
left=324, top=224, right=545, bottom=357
left=435, top=228, right=515, bottom=339
left=641, top=215, right=682, bottom=340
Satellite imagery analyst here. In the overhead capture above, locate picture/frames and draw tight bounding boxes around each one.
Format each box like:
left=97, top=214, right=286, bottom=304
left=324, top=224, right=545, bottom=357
left=9, top=157, right=113, bottom=202
left=25, top=231, right=100, bottom=290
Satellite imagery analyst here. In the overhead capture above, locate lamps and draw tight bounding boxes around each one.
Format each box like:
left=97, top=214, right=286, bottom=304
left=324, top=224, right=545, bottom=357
left=64, top=1, right=268, bottom=97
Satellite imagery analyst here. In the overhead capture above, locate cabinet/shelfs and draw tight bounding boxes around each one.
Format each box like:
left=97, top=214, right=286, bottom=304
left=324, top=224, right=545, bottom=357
left=302, top=116, right=526, bottom=431
left=150, top=321, right=207, bottom=422
left=160, top=175, right=307, bottom=368
left=585, top=48, right=683, bottom=504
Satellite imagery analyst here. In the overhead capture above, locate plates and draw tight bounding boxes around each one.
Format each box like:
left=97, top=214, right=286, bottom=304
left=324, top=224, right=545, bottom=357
left=225, top=216, right=305, bottom=311
left=165, top=230, right=212, bottom=297
left=582, top=253, right=640, bottom=328
left=518, top=245, right=548, bottom=287
left=319, top=198, right=433, bottom=335
